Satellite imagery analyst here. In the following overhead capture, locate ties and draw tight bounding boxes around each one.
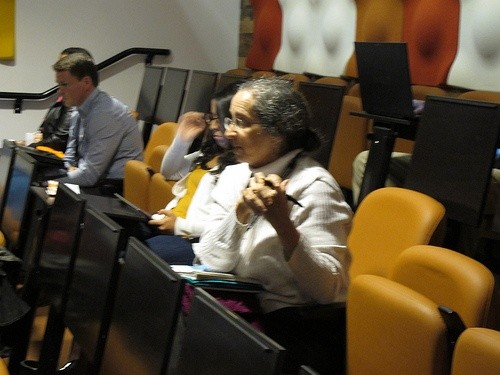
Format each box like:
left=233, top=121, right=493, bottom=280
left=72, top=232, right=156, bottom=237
left=75, top=117, right=81, bottom=166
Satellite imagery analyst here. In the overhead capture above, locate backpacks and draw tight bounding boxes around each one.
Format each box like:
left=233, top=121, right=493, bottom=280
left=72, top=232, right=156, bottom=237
left=0, top=246, right=29, bottom=359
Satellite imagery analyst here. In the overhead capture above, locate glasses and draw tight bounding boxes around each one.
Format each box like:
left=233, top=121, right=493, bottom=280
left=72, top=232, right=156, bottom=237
left=223, top=117, right=263, bottom=129
left=202, top=113, right=222, bottom=125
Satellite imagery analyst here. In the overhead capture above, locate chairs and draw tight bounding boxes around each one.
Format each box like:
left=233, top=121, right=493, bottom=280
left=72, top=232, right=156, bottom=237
left=0, top=66, right=500, bottom=375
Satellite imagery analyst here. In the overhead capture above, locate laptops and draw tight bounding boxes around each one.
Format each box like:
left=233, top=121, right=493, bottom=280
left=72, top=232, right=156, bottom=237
left=354, top=42, right=421, bottom=119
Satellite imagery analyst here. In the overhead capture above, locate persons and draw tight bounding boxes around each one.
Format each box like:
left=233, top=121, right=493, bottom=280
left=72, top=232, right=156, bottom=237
left=17, top=47, right=94, bottom=158
left=32, top=53, right=146, bottom=202
left=57, top=81, right=239, bottom=375
left=192, top=75, right=354, bottom=313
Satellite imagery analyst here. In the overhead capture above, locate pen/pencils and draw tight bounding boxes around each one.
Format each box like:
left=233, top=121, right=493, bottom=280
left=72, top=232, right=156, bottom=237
left=262, top=178, right=303, bottom=209
